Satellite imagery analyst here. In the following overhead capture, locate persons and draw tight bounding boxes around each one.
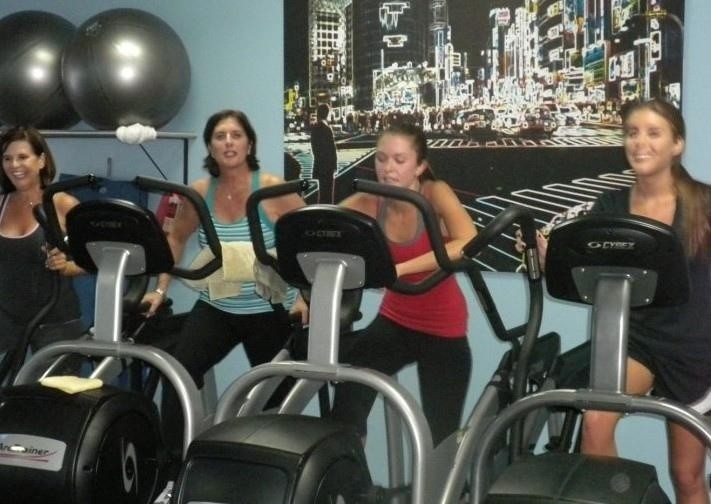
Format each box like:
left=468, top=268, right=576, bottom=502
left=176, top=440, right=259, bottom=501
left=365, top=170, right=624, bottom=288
left=140, top=108, right=308, bottom=503
left=310, top=103, right=338, bottom=204
left=0, top=125, right=89, bottom=385
left=515, top=98, right=711, bottom=504
left=333, top=122, right=478, bottom=504
left=344, top=104, right=463, bottom=135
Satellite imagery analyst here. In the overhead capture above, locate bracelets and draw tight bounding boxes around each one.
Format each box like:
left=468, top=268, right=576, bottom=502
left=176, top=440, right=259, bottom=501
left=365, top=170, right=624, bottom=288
left=154, top=288, right=166, bottom=298
